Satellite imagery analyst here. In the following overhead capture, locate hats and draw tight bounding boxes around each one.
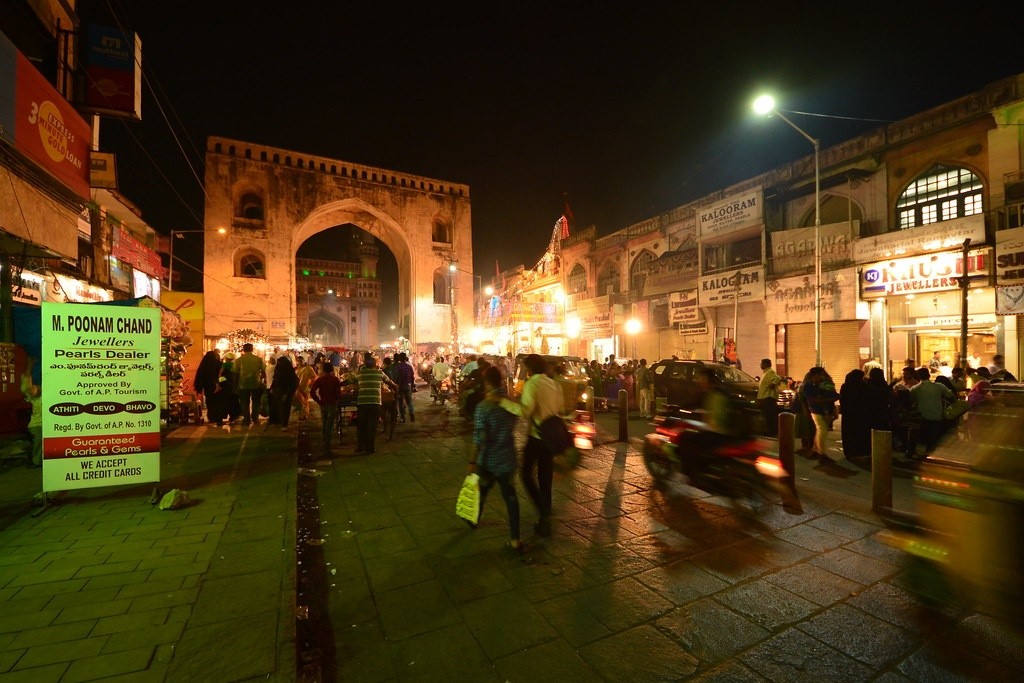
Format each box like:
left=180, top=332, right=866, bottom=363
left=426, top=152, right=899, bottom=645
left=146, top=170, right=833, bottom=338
left=226, top=353, right=235, bottom=360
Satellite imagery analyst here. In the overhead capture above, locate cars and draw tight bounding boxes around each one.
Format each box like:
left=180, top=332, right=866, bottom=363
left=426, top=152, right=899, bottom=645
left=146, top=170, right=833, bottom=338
left=514, top=354, right=590, bottom=405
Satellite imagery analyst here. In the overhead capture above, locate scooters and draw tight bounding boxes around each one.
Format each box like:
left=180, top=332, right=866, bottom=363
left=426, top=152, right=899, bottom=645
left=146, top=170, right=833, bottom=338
left=642, top=416, right=789, bottom=515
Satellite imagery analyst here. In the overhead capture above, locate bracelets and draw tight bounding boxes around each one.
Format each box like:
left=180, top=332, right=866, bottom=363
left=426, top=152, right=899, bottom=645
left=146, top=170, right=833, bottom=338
left=468, top=462, right=478, bottom=464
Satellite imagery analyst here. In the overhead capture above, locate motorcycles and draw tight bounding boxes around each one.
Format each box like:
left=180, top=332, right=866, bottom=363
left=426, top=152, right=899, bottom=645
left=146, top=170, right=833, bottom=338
left=417, top=364, right=452, bottom=405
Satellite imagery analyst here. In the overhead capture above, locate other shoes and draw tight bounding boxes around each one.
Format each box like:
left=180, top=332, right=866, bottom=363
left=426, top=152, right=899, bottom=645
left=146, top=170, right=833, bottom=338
left=354, top=447, right=364, bottom=452
left=242, top=418, right=251, bottom=424
left=298, top=416, right=307, bottom=421
left=400, top=417, right=405, bottom=423
left=410, top=413, right=415, bottom=422
left=323, top=438, right=329, bottom=450
left=280, top=426, right=289, bottom=432
left=534, top=522, right=551, bottom=537
left=809, top=451, right=830, bottom=465
left=365, top=447, right=377, bottom=455
left=208, top=417, right=236, bottom=427
left=504, top=540, right=529, bottom=555
left=460, top=515, right=478, bottom=528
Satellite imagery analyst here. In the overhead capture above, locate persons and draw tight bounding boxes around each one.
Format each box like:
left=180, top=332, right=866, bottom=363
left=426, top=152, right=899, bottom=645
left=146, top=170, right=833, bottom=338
left=193, top=346, right=487, bottom=454
left=459, top=363, right=530, bottom=555
left=758, top=358, right=782, bottom=437
left=487, top=350, right=565, bottom=539
left=890, top=350, right=1018, bottom=474
left=20, top=356, right=42, bottom=469
left=583, top=354, right=655, bottom=422
left=802, top=366, right=838, bottom=460
left=839, top=354, right=892, bottom=460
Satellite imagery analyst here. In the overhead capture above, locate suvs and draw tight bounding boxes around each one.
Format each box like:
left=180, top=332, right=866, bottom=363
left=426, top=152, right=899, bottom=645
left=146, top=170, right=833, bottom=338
left=650, top=357, right=795, bottom=413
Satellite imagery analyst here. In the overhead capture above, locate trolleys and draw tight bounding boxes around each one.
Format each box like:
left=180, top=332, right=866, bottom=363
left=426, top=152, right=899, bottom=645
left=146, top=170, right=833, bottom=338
left=336, top=384, right=399, bottom=445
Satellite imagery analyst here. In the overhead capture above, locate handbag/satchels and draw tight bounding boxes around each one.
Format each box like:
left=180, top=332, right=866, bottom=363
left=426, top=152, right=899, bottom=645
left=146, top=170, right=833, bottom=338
left=942, top=390, right=970, bottom=420
left=534, top=414, right=574, bottom=456
left=455, top=472, right=481, bottom=525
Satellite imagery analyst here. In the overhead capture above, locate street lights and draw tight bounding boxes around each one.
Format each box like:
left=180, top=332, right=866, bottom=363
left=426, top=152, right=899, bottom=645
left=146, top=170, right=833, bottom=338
left=168, top=228, right=225, bottom=291
left=450, top=265, right=482, bottom=324
left=750, top=94, right=820, bottom=370
